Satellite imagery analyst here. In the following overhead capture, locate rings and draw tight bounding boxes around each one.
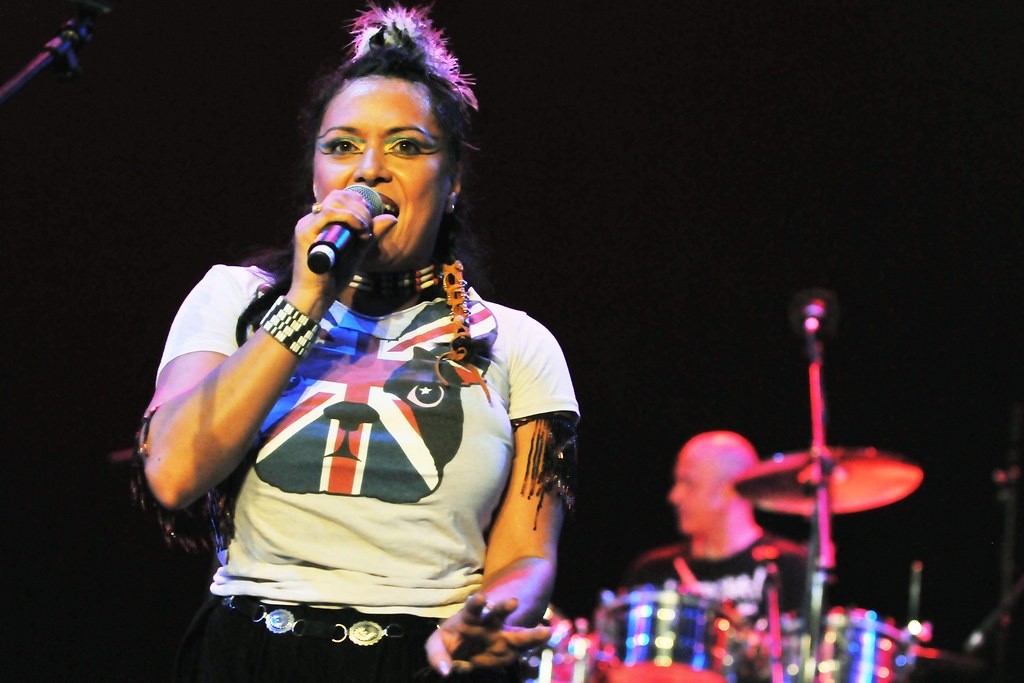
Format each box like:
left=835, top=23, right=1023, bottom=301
left=312, top=204, right=321, bottom=213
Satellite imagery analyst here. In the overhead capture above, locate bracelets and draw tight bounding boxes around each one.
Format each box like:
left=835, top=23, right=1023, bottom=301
left=259, top=296, right=321, bottom=358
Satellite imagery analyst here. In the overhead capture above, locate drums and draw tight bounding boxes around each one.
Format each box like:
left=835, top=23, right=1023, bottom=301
left=597, top=586, right=758, bottom=683
left=759, top=608, right=928, bottom=683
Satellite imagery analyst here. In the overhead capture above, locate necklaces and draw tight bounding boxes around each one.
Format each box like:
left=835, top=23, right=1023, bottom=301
left=349, top=262, right=442, bottom=294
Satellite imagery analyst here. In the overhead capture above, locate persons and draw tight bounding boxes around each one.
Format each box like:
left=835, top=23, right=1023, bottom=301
left=138, top=6, right=580, bottom=683
left=620, top=431, right=844, bottom=622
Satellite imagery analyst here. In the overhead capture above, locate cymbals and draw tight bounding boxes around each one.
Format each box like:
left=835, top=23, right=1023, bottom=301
left=730, top=448, right=923, bottom=515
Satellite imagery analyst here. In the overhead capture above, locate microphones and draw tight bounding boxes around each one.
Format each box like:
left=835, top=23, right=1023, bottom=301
left=790, top=288, right=840, bottom=338
left=306, top=185, right=383, bottom=273
